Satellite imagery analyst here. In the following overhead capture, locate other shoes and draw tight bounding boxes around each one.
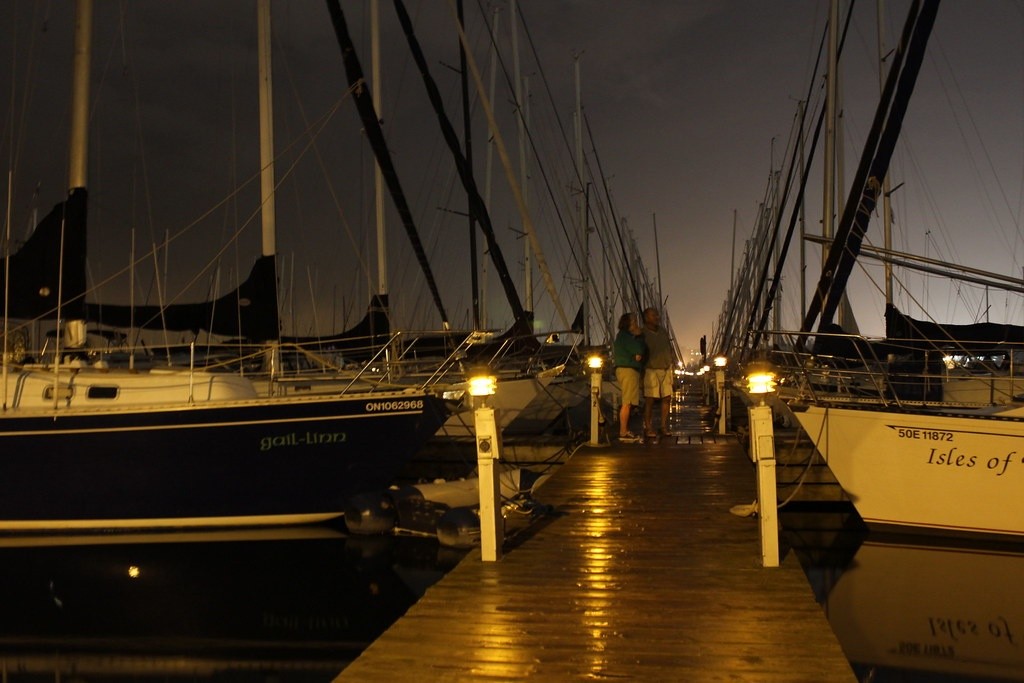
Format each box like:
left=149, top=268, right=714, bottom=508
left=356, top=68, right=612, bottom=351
left=658, top=428, right=672, bottom=437
left=644, top=427, right=657, bottom=438
left=618, top=431, right=645, bottom=443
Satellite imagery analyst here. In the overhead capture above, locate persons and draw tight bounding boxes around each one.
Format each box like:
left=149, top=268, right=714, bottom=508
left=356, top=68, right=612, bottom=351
left=614, top=312, right=646, bottom=440
left=641, top=307, right=679, bottom=438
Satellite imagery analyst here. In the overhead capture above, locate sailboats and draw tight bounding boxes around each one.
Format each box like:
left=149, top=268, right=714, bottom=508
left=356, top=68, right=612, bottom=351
left=0, top=1, right=1024, bottom=549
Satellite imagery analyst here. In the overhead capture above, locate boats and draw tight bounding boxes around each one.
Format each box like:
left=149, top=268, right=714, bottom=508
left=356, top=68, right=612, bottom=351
left=819, top=537, right=1024, bottom=683
